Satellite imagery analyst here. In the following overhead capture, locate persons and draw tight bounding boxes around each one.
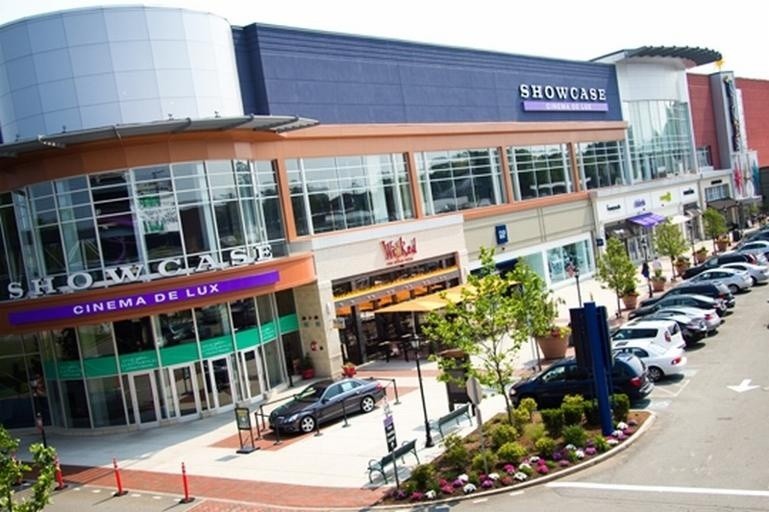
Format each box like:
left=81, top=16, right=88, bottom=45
left=653, top=257, right=661, bottom=271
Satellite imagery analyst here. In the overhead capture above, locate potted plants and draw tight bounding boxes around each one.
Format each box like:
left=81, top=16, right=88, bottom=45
left=692, top=247, right=708, bottom=262
left=675, top=256, right=690, bottom=274
left=715, top=238, right=729, bottom=251
left=534, top=325, right=572, bottom=359
left=620, top=290, right=640, bottom=308
left=650, top=276, right=667, bottom=291
left=297, top=353, right=313, bottom=380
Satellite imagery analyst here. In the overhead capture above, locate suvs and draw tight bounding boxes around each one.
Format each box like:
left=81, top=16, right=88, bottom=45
left=510, top=351, right=655, bottom=408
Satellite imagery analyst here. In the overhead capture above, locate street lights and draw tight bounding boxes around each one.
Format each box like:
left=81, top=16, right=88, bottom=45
left=571, top=264, right=583, bottom=309
left=685, top=220, right=697, bottom=266
left=734, top=201, right=743, bottom=239
left=27, top=373, right=48, bottom=454
left=409, top=332, right=435, bottom=448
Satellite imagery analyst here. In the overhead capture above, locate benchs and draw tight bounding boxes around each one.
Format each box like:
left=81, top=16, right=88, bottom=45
left=429, top=404, right=473, bottom=440
left=368, top=438, right=420, bottom=484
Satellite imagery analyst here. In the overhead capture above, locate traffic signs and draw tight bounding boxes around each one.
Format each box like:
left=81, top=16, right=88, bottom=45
left=382, top=416, right=398, bottom=451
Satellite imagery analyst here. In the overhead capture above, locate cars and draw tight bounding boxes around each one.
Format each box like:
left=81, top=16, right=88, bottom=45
left=610, top=223, right=769, bottom=384
left=268, top=375, right=385, bottom=437
